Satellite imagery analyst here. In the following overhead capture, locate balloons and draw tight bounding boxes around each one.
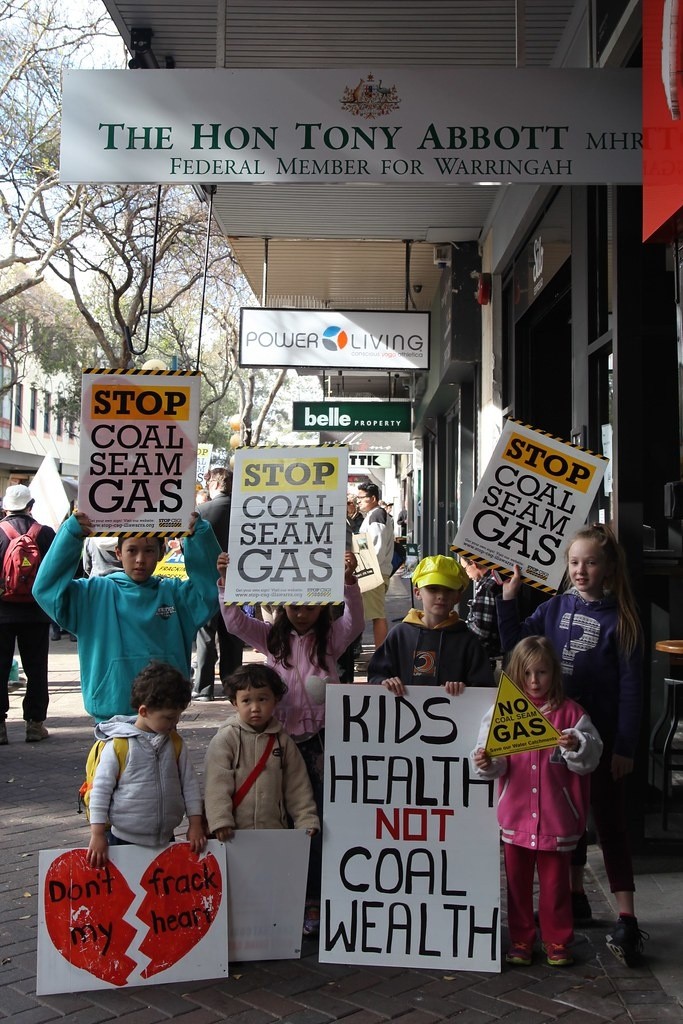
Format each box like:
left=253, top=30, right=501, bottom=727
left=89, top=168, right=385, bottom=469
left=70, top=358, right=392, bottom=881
left=229, top=434, right=241, bottom=448
left=230, top=413, right=242, bottom=431
left=230, top=455, right=235, bottom=467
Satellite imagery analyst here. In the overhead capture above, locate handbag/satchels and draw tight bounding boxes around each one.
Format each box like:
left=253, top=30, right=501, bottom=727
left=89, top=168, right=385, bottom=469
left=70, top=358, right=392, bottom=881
left=350, top=532, right=384, bottom=594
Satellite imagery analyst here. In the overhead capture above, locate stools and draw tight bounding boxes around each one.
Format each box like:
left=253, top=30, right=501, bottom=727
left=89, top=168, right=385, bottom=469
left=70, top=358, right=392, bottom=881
left=649, top=639, right=683, bottom=831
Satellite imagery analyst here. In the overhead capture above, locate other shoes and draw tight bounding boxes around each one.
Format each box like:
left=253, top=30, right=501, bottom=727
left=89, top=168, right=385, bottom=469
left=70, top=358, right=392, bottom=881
left=0, top=721, right=9, bottom=746
left=51, top=633, right=62, bottom=641
left=70, top=634, right=77, bottom=642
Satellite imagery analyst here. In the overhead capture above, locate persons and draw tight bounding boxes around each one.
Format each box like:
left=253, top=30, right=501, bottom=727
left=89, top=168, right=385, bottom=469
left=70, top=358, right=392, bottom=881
left=333, top=483, right=409, bottom=683
left=85, top=663, right=208, bottom=869
left=168, top=466, right=278, bottom=704
left=32, top=511, right=226, bottom=846
left=495, top=523, right=652, bottom=970
left=366, top=554, right=491, bottom=697
left=204, top=663, right=321, bottom=843
left=470, top=634, right=603, bottom=966
left=460, top=555, right=502, bottom=672
left=215, top=550, right=365, bottom=938
left=0, top=483, right=125, bottom=746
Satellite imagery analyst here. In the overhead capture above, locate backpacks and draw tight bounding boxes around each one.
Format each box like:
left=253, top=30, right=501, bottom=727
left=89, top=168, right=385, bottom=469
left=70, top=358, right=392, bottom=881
left=0, top=518, right=45, bottom=605
left=78, top=736, right=129, bottom=831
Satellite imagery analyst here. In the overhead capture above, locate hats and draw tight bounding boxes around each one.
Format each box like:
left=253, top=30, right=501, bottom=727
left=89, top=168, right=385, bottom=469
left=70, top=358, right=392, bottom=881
left=3, top=483, right=33, bottom=511
left=412, top=554, right=470, bottom=594
left=386, top=502, right=394, bottom=506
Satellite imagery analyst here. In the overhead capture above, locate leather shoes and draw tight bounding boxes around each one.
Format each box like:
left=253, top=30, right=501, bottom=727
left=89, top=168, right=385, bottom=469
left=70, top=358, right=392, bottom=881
left=191, top=691, right=214, bottom=702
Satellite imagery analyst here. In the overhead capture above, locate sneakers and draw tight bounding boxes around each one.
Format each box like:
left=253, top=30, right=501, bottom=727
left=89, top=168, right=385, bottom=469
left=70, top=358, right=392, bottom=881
left=540, top=940, right=569, bottom=966
left=605, top=914, right=651, bottom=968
left=24, top=720, right=49, bottom=742
left=504, top=942, right=533, bottom=964
left=533, top=892, right=593, bottom=927
